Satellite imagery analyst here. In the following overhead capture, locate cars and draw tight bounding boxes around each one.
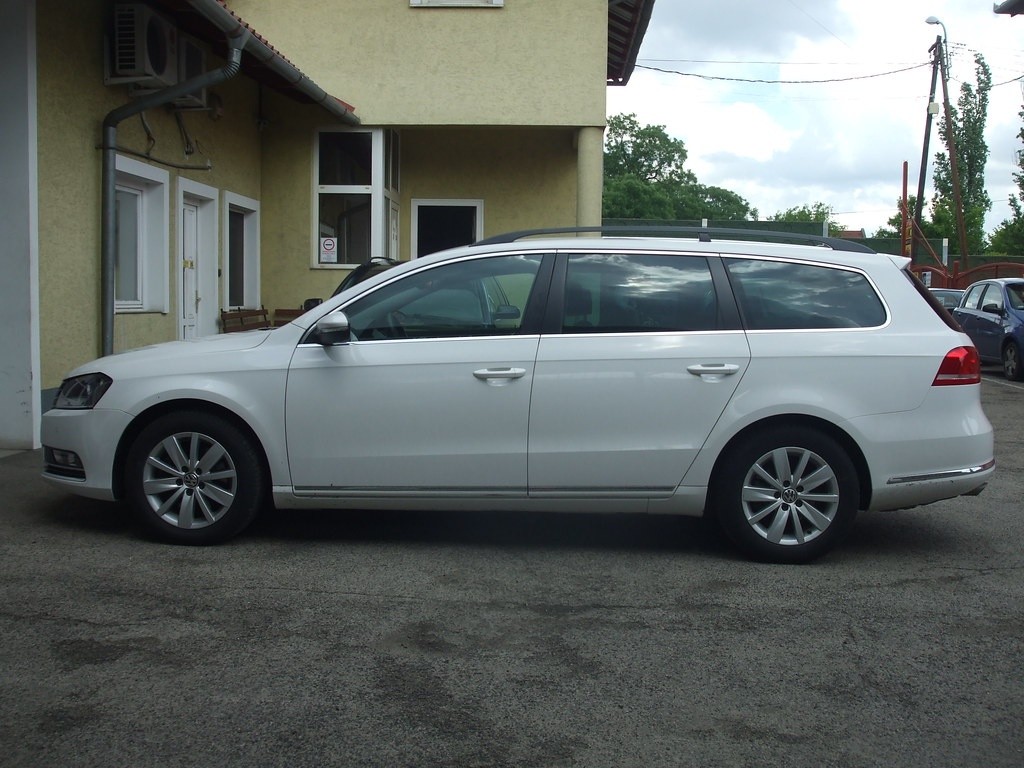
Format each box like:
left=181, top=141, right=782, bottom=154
left=924, top=287, right=969, bottom=316
left=951, top=277, right=1024, bottom=383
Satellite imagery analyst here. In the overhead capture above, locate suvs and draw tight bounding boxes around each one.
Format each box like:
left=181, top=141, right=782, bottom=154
left=38, top=223, right=997, bottom=568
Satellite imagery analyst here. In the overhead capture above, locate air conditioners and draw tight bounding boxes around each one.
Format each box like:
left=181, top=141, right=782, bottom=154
left=104, top=2, right=177, bottom=87
left=155, top=30, right=206, bottom=108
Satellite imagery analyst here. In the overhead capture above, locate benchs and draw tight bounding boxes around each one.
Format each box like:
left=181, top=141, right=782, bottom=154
left=274, top=308, right=306, bottom=326
left=221, top=305, right=271, bottom=333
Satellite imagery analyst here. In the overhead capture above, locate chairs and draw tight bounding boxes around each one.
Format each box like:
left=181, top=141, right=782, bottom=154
left=566, top=286, right=595, bottom=329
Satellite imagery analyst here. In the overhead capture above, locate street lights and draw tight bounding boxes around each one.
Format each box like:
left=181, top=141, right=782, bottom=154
left=924, top=14, right=969, bottom=270
